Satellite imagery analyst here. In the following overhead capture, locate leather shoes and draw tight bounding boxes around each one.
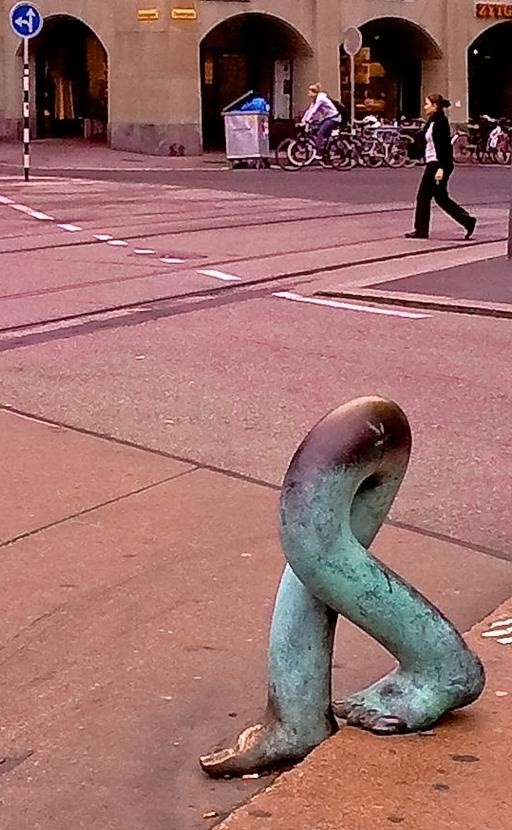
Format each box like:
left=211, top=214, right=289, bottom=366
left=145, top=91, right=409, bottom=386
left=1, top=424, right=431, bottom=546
left=464, top=216, right=477, bottom=239
left=405, top=230, right=429, bottom=238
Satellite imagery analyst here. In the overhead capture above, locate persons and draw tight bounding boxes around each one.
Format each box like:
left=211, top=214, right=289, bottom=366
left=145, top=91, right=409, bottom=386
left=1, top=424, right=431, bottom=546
left=300, top=83, right=342, bottom=160
left=405, top=94, right=477, bottom=239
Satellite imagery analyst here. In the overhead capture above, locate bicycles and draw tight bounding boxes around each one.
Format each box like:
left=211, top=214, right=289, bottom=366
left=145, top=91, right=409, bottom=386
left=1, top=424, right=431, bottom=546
left=451, top=114, right=512, bottom=166
left=272, top=114, right=418, bottom=170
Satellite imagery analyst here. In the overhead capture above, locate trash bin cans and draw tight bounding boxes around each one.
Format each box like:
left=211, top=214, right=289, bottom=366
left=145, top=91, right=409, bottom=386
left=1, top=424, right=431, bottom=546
left=221, top=90, right=271, bottom=169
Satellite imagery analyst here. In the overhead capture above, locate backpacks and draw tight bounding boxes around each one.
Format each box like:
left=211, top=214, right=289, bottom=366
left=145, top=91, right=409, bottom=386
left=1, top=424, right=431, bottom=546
left=328, top=95, right=345, bottom=113
